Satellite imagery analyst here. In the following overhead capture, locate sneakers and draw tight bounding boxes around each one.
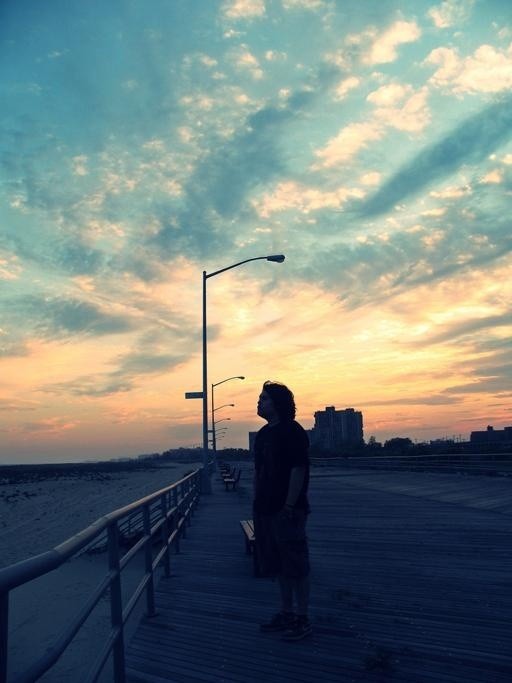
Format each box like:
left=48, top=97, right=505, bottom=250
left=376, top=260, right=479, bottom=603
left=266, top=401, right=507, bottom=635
left=262, top=613, right=312, bottom=640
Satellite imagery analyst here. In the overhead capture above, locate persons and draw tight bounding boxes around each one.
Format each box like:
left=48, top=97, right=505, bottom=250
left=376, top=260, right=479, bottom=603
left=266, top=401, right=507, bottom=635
left=253, top=380, right=312, bottom=642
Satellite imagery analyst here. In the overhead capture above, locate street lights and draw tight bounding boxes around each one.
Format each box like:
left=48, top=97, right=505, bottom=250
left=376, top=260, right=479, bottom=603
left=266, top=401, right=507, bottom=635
left=208, top=374, right=249, bottom=468
left=202, top=253, right=286, bottom=495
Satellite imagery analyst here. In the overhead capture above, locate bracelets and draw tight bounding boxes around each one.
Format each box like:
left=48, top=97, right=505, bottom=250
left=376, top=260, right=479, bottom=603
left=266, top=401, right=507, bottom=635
left=283, top=502, right=293, bottom=508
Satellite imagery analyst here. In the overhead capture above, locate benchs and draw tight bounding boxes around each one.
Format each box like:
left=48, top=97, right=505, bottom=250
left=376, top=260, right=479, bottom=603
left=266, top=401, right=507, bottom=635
left=219, top=462, right=241, bottom=488
left=239, top=518, right=270, bottom=577
